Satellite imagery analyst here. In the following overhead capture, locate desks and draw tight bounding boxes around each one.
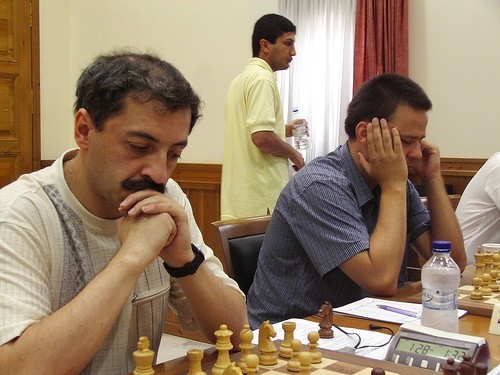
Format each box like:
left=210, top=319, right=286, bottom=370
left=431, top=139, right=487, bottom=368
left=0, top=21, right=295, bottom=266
left=122, top=262, right=500, bottom=375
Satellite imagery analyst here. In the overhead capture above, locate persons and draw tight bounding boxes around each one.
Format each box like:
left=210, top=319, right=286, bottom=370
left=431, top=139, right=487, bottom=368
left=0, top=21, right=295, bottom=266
left=1, top=50, right=250, bottom=375
left=454, top=151, right=500, bottom=264
left=244, top=73, right=468, bottom=331
left=220, top=13, right=310, bottom=225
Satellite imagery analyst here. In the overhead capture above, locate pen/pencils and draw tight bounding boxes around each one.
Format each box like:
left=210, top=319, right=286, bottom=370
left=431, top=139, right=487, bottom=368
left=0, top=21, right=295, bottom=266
left=377, top=304, right=419, bottom=318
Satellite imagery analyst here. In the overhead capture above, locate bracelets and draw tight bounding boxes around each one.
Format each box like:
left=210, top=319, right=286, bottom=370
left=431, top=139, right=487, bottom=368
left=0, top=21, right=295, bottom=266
left=163, top=243, right=205, bottom=278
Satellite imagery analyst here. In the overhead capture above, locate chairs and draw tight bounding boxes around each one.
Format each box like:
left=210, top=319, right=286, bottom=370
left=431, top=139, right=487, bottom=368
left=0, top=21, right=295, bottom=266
left=210, top=214, right=272, bottom=304
left=408, top=194, right=462, bottom=282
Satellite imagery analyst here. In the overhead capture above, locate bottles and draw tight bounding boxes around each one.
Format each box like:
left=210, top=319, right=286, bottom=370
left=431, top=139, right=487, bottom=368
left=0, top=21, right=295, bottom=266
left=421, top=241, right=460, bottom=334
left=292, top=109, right=310, bottom=150
